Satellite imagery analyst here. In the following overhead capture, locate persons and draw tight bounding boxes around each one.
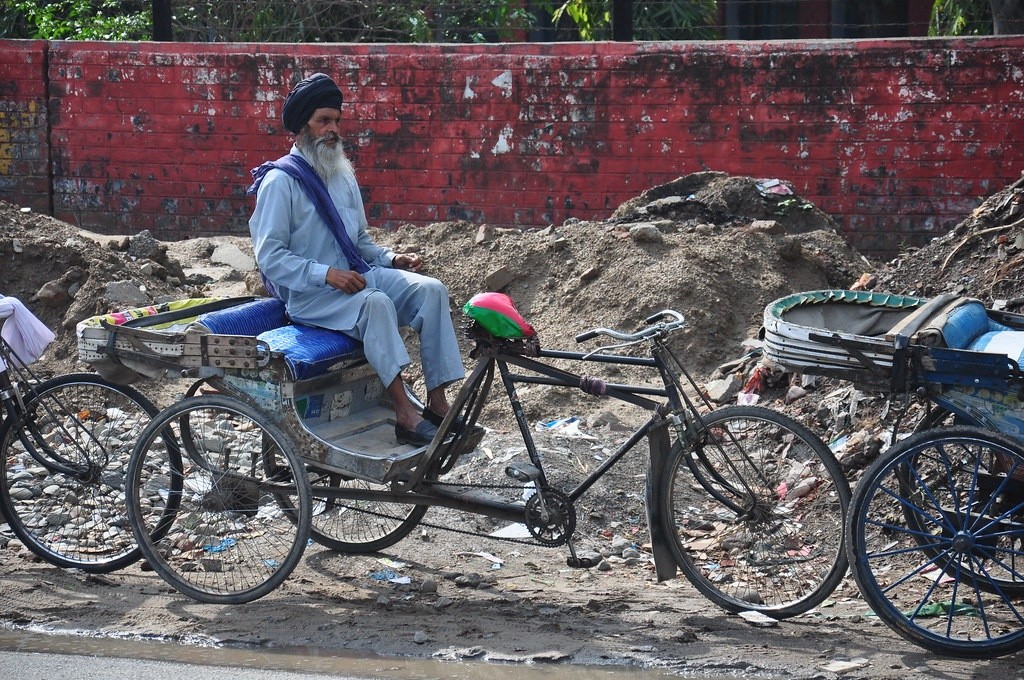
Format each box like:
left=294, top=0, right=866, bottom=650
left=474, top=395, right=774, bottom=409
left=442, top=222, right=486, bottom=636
left=248, top=72, right=483, bottom=446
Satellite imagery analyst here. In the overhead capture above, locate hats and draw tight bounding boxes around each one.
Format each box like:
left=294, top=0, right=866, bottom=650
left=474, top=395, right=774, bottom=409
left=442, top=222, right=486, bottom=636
left=282, top=72, right=343, bottom=137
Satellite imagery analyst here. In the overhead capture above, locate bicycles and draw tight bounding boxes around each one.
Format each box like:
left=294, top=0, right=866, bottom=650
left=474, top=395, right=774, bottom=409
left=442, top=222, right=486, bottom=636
left=0, top=296, right=184, bottom=574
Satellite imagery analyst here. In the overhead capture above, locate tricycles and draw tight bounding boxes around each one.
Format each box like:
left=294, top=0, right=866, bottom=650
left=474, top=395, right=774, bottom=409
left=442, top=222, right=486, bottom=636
left=758, top=289, right=1024, bottom=661
left=76, top=293, right=852, bottom=619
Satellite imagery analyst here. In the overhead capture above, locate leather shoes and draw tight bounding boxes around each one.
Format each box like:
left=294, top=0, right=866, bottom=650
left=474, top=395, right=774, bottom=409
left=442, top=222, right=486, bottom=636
left=422, top=406, right=483, bottom=434
left=396, top=420, right=450, bottom=446
